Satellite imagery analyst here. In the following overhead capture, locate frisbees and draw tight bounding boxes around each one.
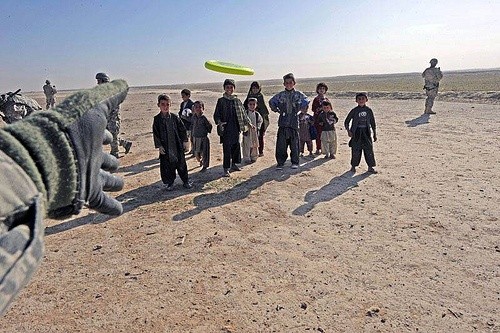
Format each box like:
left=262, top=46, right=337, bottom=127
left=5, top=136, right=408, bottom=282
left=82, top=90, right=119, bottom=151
left=205, top=60, right=254, bottom=75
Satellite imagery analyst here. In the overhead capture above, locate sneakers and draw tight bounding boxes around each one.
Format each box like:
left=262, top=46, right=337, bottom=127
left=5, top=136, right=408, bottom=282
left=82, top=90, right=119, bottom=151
left=317, top=149, right=321, bottom=154
left=259, top=153, right=264, bottom=157
left=224, top=170, right=229, bottom=177
left=185, top=179, right=193, bottom=188
left=231, top=166, right=239, bottom=171
left=276, top=164, right=284, bottom=169
left=368, top=168, right=376, bottom=173
left=301, top=154, right=306, bottom=159
left=251, top=157, right=256, bottom=162
left=351, top=168, right=356, bottom=172
left=125, top=142, right=132, bottom=153
left=245, top=160, right=251, bottom=164
left=166, top=184, right=174, bottom=190
left=309, top=154, right=316, bottom=158
left=292, top=163, right=298, bottom=168
left=110, top=153, right=120, bottom=158
left=324, top=156, right=328, bottom=159
left=331, top=155, right=335, bottom=158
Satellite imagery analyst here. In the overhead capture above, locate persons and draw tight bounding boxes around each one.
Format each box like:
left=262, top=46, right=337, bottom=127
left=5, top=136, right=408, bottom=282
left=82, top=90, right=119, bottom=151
left=0, top=78, right=130, bottom=312
left=268, top=72, right=310, bottom=170
left=311, top=82, right=334, bottom=154
left=0, top=87, right=43, bottom=127
left=191, top=100, right=213, bottom=172
left=213, top=78, right=256, bottom=177
left=150, top=94, right=192, bottom=192
left=419, top=57, right=442, bottom=117
left=317, top=100, right=339, bottom=158
left=241, top=97, right=263, bottom=162
left=342, top=91, right=379, bottom=175
left=40, top=79, right=58, bottom=111
left=176, top=88, right=193, bottom=154
left=298, top=105, right=314, bottom=157
left=242, top=80, right=270, bottom=157
left=94, top=71, right=132, bottom=157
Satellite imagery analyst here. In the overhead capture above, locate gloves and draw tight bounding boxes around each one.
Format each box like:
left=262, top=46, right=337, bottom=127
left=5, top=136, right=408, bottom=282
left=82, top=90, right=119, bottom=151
left=0, top=80, right=128, bottom=219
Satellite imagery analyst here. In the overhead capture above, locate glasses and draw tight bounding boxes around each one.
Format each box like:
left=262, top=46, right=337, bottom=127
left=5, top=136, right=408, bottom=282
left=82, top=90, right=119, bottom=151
left=252, top=86, right=258, bottom=89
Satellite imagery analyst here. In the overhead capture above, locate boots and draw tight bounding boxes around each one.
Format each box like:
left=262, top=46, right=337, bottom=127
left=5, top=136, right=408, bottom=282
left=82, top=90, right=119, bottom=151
left=425, top=106, right=436, bottom=114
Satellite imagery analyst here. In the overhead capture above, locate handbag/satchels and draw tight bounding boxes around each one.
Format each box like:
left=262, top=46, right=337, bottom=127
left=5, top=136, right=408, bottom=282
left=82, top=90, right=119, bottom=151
left=217, top=125, right=224, bottom=136
left=310, top=125, right=317, bottom=140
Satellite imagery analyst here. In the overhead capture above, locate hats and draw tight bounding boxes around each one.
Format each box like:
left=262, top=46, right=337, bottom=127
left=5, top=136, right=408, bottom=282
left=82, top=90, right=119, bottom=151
left=283, top=73, right=294, bottom=81
left=46, top=80, right=50, bottom=84
left=224, top=79, right=235, bottom=89
left=356, top=92, right=367, bottom=99
left=250, top=82, right=259, bottom=91
left=248, top=98, right=257, bottom=101
left=300, top=99, right=310, bottom=108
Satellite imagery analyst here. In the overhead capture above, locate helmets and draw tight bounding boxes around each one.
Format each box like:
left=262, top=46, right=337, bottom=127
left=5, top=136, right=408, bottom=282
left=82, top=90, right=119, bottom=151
left=96, top=73, right=110, bottom=82
left=430, top=59, right=437, bottom=63
